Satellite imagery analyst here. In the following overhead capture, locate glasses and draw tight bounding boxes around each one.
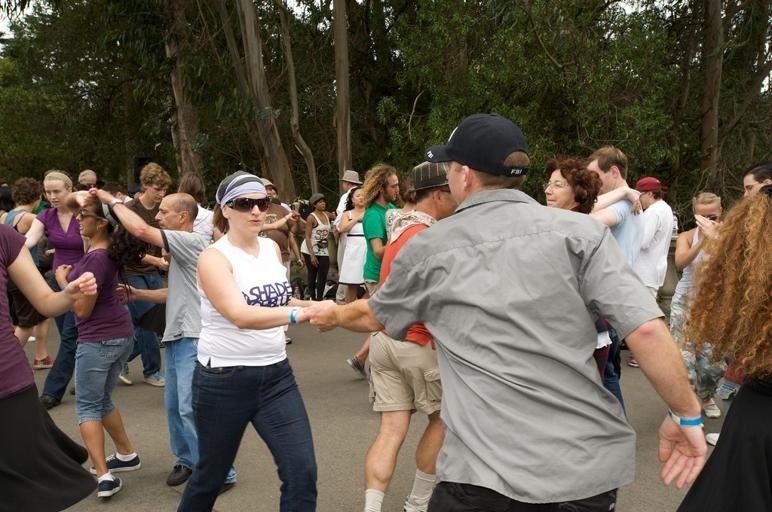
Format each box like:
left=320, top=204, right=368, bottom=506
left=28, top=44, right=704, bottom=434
left=224, top=198, right=270, bottom=212
left=544, top=178, right=571, bottom=190
left=708, top=214, right=723, bottom=222
left=78, top=208, right=103, bottom=221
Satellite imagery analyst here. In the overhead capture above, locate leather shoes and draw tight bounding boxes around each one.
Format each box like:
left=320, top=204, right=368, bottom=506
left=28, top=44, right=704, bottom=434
left=165, top=464, right=192, bottom=487
left=217, top=480, right=234, bottom=496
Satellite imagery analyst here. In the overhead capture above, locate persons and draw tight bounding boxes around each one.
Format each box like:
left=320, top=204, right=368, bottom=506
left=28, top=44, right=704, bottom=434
left=401, top=174, right=413, bottom=209
left=673, top=194, right=771, bottom=511
left=21, top=171, right=87, bottom=413
left=332, top=169, right=363, bottom=309
left=0, top=223, right=97, bottom=512
left=347, top=163, right=412, bottom=380
left=103, top=184, right=133, bottom=204
left=743, top=159, right=772, bottom=200
left=667, top=193, right=728, bottom=420
left=258, top=178, right=305, bottom=344
left=174, top=172, right=225, bottom=243
left=271, top=187, right=293, bottom=215
left=79, top=170, right=98, bottom=191
left=55, top=193, right=141, bottom=498
left=585, top=147, right=642, bottom=428
left=175, top=173, right=328, bottom=512
left=1, top=178, right=16, bottom=212
left=108, top=162, right=172, bottom=388
left=0, top=177, right=57, bottom=370
left=288, top=205, right=313, bottom=299
left=626, top=175, right=673, bottom=368
left=362, top=161, right=448, bottom=512
left=337, top=186, right=370, bottom=300
left=541, top=160, right=611, bottom=387
left=89, top=186, right=237, bottom=497
left=301, top=194, right=331, bottom=300
left=758, top=185, right=771, bottom=199
left=310, top=114, right=708, bottom=512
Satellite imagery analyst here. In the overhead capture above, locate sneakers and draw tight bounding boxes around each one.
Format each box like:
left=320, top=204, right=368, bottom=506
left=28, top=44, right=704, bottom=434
left=142, top=372, right=164, bottom=390
left=346, top=354, right=367, bottom=378
left=701, top=395, right=721, bottom=418
left=97, top=477, right=122, bottom=497
left=284, top=331, right=292, bottom=344
left=88, top=453, right=141, bottom=474
left=717, top=379, right=741, bottom=400
left=624, top=357, right=639, bottom=368
left=37, top=391, right=60, bottom=411
left=32, top=356, right=56, bottom=369
left=705, top=432, right=721, bottom=446
left=117, top=363, right=134, bottom=385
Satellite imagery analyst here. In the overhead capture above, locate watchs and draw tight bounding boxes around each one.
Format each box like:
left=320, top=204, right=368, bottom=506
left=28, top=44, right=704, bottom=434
left=109, top=199, right=123, bottom=208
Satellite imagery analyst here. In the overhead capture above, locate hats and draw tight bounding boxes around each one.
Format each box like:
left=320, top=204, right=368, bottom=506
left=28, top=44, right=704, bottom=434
left=424, top=112, right=533, bottom=177
left=310, top=192, right=323, bottom=206
left=636, top=175, right=661, bottom=191
left=339, top=168, right=365, bottom=187
left=260, top=176, right=274, bottom=187
left=409, top=159, right=449, bottom=193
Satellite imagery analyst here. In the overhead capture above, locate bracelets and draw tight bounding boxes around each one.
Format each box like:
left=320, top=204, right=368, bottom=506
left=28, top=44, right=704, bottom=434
left=668, top=408, right=706, bottom=425
left=289, top=305, right=299, bottom=323
